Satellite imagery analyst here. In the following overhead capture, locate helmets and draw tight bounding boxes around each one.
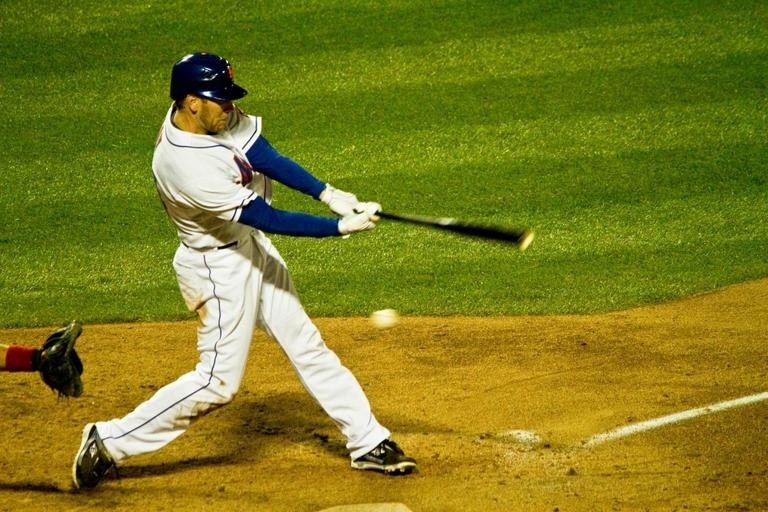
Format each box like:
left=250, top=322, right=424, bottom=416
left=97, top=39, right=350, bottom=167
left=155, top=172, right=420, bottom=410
left=169, top=50, right=249, bottom=103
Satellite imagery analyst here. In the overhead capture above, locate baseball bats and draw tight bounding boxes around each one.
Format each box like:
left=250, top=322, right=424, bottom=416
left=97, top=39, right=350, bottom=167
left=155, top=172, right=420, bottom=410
left=374, top=207, right=533, bottom=252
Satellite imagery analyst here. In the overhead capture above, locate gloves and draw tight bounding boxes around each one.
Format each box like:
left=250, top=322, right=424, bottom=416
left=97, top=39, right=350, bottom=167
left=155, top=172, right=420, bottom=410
left=321, top=183, right=359, bottom=216
left=337, top=201, right=382, bottom=235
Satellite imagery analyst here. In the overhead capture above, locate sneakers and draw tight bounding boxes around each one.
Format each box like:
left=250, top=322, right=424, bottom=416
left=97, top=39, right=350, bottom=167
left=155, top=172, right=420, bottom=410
left=350, top=439, right=418, bottom=476
left=71, top=421, right=115, bottom=494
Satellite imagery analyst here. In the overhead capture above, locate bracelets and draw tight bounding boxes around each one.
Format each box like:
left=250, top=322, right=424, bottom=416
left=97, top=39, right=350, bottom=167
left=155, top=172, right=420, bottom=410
left=5, top=342, right=37, bottom=375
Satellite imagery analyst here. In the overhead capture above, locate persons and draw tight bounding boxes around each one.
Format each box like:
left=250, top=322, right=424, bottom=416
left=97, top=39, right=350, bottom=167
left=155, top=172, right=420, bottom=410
left=69, top=59, right=420, bottom=495
left=0, top=320, right=85, bottom=400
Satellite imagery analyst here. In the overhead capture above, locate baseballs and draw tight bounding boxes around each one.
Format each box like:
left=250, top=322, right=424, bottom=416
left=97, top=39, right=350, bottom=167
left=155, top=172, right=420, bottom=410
left=372, top=308, right=399, bottom=330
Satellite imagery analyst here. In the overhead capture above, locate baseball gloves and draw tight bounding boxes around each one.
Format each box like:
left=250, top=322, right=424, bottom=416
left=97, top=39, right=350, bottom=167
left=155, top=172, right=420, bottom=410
left=34, top=320, right=86, bottom=398
